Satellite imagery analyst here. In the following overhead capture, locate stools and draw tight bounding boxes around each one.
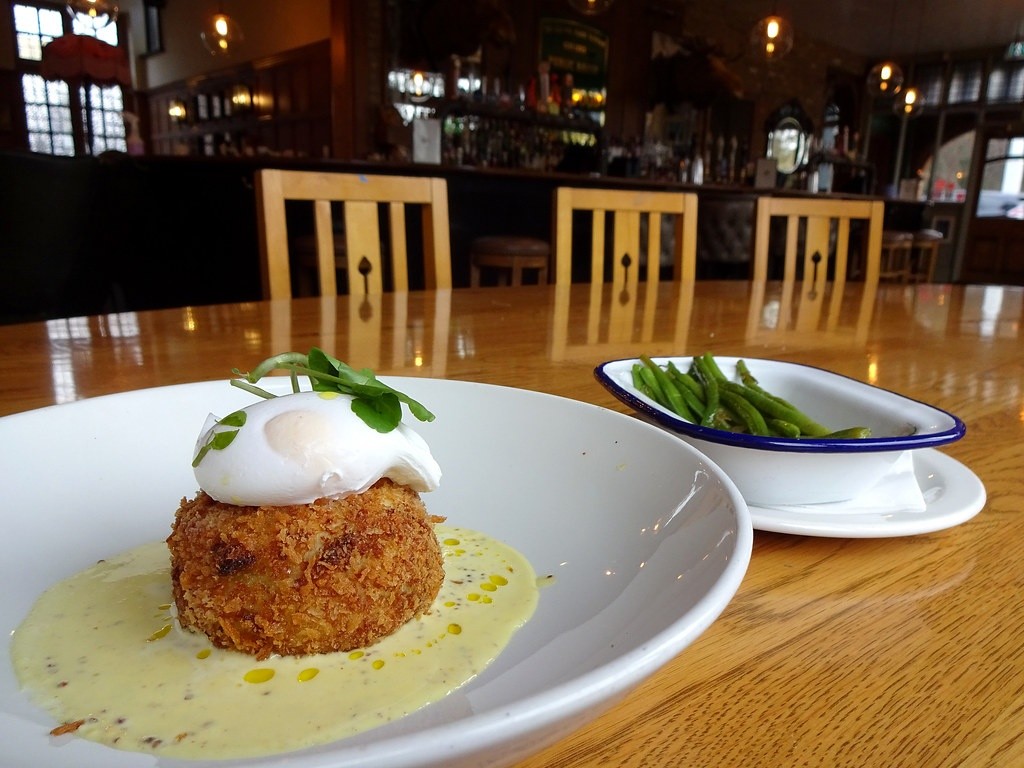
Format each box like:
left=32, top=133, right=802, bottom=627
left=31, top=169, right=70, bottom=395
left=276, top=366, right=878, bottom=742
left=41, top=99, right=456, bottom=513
left=299, top=233, right=391, bottom=297
left=469, top=238, right=548, bottom=289
left=848, top=226, right=946, bottom=283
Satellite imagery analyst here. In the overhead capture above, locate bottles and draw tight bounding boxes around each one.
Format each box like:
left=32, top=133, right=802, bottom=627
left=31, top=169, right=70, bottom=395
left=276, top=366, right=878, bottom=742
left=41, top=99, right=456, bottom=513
left=387, top=66, right=857, bottom=193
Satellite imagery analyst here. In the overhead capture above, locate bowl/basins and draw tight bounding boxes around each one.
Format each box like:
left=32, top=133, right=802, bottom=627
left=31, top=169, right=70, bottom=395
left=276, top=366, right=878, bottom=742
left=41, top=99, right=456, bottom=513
left=597, top=350, right=970, bottom=509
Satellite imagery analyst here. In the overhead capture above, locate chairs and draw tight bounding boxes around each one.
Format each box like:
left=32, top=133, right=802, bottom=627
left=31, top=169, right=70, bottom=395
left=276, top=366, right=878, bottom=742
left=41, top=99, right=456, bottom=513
left=554, top=186, right=698, bottom=284
left=752, top=197, right=885, bottom=283
left=254, top=167, right=454, bottom=301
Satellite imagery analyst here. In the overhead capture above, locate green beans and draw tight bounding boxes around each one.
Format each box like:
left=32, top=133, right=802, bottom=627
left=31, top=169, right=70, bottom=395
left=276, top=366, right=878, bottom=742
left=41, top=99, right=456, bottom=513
left=630, top=350, right=872, bottom=439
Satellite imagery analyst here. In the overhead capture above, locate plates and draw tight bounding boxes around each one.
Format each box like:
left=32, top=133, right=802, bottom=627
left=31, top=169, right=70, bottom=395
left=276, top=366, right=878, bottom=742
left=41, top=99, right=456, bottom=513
left=0, top=371, right=760, bottom=767
left=747, top=451, right=987, bottom=541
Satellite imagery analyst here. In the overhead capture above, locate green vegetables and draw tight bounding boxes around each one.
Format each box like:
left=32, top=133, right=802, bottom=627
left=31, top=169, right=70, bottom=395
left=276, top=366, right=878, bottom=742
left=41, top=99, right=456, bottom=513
left=227, top=346, right=435, bottom=433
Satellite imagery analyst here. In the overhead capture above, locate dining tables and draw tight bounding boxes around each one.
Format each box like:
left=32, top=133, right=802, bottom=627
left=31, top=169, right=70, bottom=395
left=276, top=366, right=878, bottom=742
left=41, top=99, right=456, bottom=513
left=0, top=283, right=1024, bottom=765
